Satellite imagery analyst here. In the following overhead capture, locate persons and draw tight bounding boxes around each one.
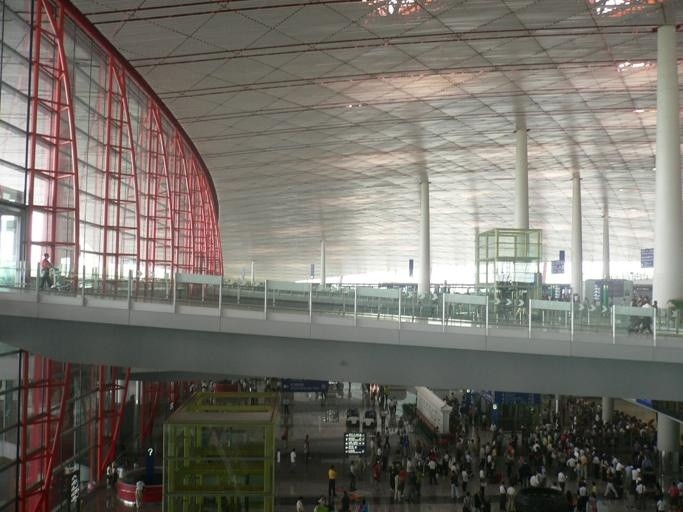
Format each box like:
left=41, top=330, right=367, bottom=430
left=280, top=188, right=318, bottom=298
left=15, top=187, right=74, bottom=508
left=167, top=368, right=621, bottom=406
left=38, top=252, right=59, bottom=288
left=134, top=477, right=147, bottom=508
left=625, top=294, right=658, bottom=333
left=104, top=454, right=139, bottom=488
left=542, top=291, right=614, bottom=307
left=169, top=378, right=682, bottom=512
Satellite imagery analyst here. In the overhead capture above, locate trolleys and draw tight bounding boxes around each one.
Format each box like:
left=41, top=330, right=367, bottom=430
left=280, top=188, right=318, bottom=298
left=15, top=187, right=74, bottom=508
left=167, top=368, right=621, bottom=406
left=50, top=267, right=71, bottom=292
left=628, top=317, right=644, bottom=335
